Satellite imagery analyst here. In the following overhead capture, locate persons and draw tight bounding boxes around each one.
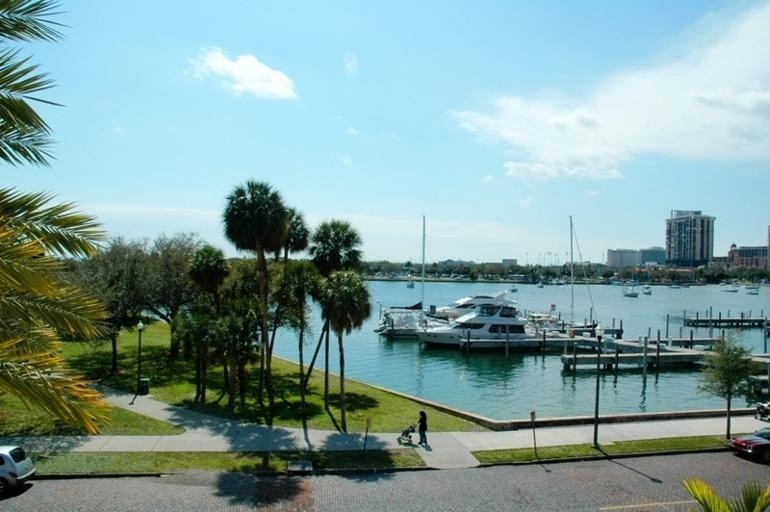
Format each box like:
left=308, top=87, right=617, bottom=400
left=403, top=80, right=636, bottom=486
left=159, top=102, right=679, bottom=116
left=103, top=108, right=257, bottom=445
left=417, top=411, right=428, bottom=444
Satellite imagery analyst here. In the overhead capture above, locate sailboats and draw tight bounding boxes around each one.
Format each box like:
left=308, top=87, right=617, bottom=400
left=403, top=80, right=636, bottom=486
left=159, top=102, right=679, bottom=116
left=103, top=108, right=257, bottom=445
left=374, top=215, right=599, bottom=347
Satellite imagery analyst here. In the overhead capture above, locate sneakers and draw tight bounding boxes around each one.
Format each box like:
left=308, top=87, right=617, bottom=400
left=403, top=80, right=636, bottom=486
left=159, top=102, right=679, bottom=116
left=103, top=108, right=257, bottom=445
left=418, top=442, right=421, bottom=443
left=424, top=441, right=426, bottom=443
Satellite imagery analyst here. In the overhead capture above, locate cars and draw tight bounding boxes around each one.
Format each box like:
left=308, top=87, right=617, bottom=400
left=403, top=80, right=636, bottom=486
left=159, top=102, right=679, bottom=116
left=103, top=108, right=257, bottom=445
left=728, top=427, right=770, bottom=461
left=0, top=445, right=34, bottom=492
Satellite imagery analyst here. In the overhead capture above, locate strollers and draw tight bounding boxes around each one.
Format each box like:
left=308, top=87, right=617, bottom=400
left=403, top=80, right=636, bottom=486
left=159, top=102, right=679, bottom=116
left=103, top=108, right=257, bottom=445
left=754, top=403, right=770, bottom=421
left=398, top=424, right=418, bottom=445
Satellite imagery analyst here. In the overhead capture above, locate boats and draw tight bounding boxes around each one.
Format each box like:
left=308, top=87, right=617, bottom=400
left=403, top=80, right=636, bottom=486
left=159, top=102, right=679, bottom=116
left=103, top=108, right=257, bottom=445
left=623, top=288, right=639, bottom=297
left=746, top=285, right=759, bottom=294
left=670, top=284, right=681, bottom=289
left=623, top=280, right=639, bottom=286
left=641, top=285, right=652, bottom=295
left=723, top=286, right=736, bottom=290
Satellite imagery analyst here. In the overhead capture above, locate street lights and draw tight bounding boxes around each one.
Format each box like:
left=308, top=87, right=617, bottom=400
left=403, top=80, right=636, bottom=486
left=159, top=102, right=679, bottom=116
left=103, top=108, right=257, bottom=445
left=136, top=321, right=144, bottom=395
